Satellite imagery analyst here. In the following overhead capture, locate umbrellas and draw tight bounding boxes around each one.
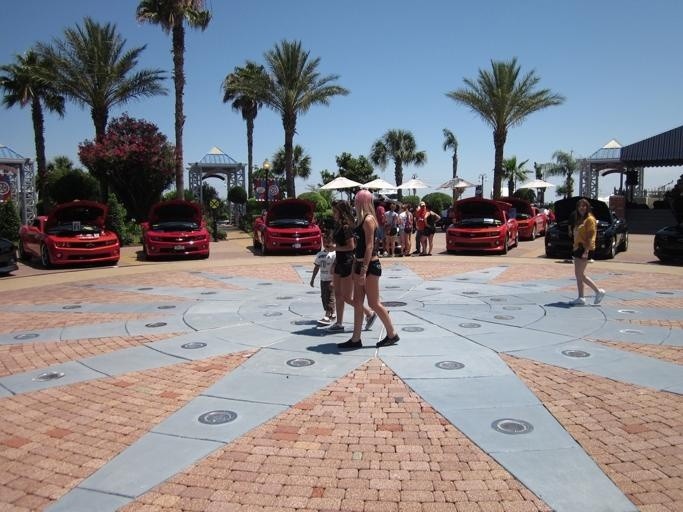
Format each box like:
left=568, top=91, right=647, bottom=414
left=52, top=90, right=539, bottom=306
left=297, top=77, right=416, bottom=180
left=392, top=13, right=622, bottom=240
left=437, top=176, right=475, bottom=201
left=395, top=175, right=430, bottom=210
left=358, top=177, right=396, bottom=193
left=318, top=176, right=362, bottom=200
left=518, top=178, right=554, bottom=189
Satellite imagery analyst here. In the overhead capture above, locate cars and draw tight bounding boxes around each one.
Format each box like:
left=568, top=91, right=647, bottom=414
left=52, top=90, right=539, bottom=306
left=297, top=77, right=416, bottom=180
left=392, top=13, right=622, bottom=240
left=445, top=196, right=521, bottom=254
left=373, top=192, right=405, bottom=202
left=0, top=236, right=21, bottom=276
left=501, top=196, right=547, bottom=242
left=17, top=198, right=121, bottom=268
left=650, top=191, right=682, bottom=265
left=251, top=199, right=323, bottom=257
left=540, top=195, right=631, bottom=261
left=137, top=198, right=212, bottom=262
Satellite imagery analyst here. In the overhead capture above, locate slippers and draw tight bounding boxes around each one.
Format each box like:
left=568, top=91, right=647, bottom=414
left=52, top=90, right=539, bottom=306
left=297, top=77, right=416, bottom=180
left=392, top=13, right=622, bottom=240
left=377, top=248, right=433, bottom=257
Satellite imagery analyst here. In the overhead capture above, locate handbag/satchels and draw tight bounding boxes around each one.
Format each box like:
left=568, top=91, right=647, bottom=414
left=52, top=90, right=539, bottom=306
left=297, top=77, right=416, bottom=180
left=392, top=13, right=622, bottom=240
left=389, top=227, right=397, bottom=236
left=404, top=227, right=412, bottom=233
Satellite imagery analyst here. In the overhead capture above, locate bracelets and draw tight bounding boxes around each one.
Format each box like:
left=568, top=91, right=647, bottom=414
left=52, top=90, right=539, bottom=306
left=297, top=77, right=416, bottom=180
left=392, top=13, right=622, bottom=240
left=359, top=264, right=368, bottom=273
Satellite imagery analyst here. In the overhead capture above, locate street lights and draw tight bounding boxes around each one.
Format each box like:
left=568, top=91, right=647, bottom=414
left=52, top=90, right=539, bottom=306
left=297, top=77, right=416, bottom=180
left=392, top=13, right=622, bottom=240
left=262, top=157, right=271, bottom=203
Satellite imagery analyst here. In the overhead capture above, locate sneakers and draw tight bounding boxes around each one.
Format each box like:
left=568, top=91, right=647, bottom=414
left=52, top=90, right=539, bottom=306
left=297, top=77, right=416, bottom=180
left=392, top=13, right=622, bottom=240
left=328, top=313, right=337, bottom=320
left=594, top=288, right=606, bottom=303
left=568, top=297, right=586, bottom=306
left=376, top=333, right=400, bottom=347
left=324, top=322, right=345, bottom=333
left=364, top=310, right=377, bottom=330
left=317, top=317, right=331, bottom=324
left=337, top=339, right=363, bottom=350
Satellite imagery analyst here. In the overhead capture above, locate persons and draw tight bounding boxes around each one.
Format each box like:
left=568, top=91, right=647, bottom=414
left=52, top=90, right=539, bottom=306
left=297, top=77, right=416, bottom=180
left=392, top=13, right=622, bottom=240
left=336, top=189, right=399, bottom=348
left=374, top=200, right=442, bottom=257
left=539, top=205, right=555, bottom=227
left=323, top=198, right=376, bottom=333
left=568, top=198, right=605, bottom=305
left=309, top=239, right=336, bottom=325
